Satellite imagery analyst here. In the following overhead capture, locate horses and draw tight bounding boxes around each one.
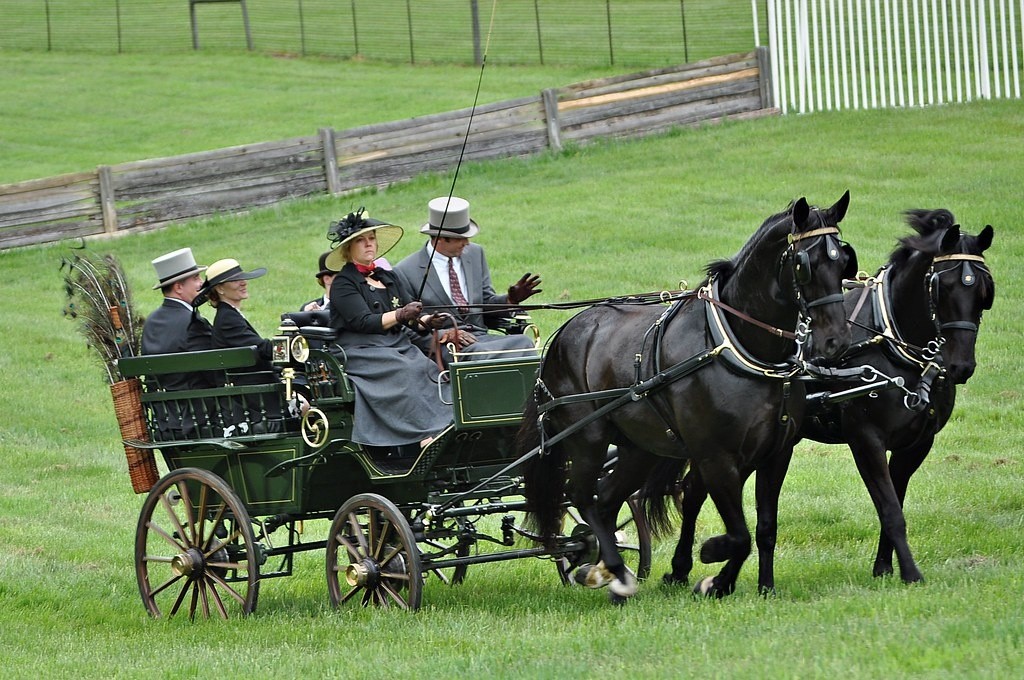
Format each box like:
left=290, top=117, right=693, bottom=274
left=515, top=190, right=850, bottom=602
left=666, top=209, right=996, bottom=587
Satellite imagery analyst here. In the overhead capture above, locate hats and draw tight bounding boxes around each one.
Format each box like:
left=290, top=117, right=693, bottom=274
left=315, top=252, right=339, bottom=276
left=191, top=258, right=267, bottom=307
left=324, top=207, right=403, bottom=271
left=152, top=247, right=208, bottom=289
left=421, top=196, right=478, bottom=237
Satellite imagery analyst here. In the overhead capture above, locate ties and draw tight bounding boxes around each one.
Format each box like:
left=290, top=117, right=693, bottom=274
left=448, top=258, right=468, bottom=312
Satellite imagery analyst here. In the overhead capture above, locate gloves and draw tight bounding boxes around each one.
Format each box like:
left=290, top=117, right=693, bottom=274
left=507, top=273, right=543, bottom=305
left=426, top=311, right=454, bottom=330
left=445, top=329, right=478, bottom=350
left=395, top=302, right=423, bottom=323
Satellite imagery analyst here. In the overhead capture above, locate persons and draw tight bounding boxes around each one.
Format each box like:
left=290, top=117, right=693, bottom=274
left=141, top=247, right=213, bottom=435
left=300, top=252, right=336, bottom=311
left=396, top=196, right=543, bottom=367
left=191, top=259, right=314, bottom=417
left=324, top=210, right=454, bottom=448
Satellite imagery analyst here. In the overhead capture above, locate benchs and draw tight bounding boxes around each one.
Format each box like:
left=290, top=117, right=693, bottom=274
left=117, top=343, right=306, bottom=449
left=287, top=309, right=524, bottom=406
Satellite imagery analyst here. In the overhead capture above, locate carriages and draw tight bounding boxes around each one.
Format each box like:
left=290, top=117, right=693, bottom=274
left=116, top=187, right=995, bottom=627
